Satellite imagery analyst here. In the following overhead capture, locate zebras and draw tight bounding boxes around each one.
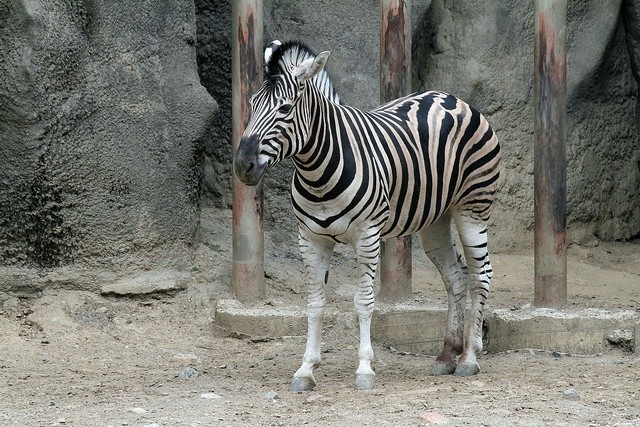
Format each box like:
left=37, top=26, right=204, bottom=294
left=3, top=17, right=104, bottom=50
left=234, top=39, right=503, bottom=392
